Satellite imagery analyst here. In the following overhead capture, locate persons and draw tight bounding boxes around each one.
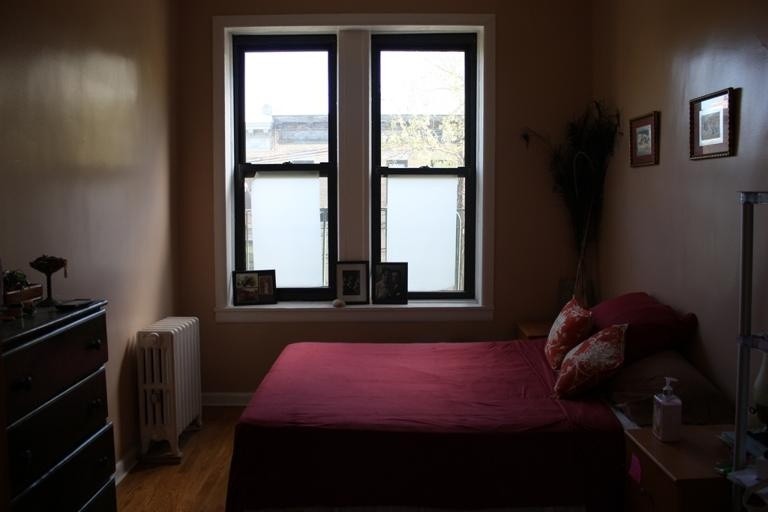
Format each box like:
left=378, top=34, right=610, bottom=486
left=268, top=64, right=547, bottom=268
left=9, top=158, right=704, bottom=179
left=374, top=267, right=391, bottom=301
left=387, top=269, right=403, bottom=301
left=343, top=277, right=359, bottom=296
left=343, top=272, right=354, bottom=289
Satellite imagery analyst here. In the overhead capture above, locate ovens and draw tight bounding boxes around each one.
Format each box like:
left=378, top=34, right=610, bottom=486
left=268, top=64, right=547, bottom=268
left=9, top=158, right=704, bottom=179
left=223, top=337, right=734, bottom=511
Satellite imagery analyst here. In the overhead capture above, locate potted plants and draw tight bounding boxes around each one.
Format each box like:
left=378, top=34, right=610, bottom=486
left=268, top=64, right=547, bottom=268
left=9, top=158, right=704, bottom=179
left=333, top=258, right=370, bottom=305
left=687, top=84, right=736, bottom=162
left=371, top=261, right=409, bottom=306
left=627, top=108, right=661, bottom=168
left=231, top=268, right=277, bottom=306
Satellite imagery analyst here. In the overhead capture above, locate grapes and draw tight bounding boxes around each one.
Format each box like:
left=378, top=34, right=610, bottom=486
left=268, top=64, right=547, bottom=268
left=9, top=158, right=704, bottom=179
left=619, top=422, right=766, bottom=511
left=516, top=317, right=554, bottom=339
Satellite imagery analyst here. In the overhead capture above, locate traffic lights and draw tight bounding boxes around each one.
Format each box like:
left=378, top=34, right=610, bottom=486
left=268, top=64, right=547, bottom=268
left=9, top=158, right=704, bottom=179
left=581, top=290, right=699, bottom=364
left=549, top=320, right=630, bottom=402
left=543, top=293, right=594, bottom=377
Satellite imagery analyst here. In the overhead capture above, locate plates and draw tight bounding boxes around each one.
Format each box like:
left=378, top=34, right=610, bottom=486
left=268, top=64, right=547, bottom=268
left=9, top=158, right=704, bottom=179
left=0, top=298, right=120, bottom=512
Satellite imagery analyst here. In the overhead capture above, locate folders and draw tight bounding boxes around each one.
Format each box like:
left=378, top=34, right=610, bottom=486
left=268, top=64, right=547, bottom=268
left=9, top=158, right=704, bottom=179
left=133, top=313, right=205, bottom=468
